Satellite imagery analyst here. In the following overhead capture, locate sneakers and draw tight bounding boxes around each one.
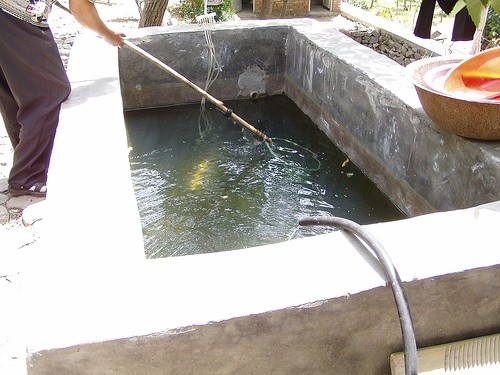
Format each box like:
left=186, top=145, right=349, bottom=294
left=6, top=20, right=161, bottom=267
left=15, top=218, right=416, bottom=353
left=10, top=174, right=48, bottom=197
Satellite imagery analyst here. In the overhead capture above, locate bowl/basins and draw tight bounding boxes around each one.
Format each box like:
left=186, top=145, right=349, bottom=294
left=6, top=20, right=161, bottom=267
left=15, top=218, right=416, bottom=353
left=404, top=46, right=500, bottom=141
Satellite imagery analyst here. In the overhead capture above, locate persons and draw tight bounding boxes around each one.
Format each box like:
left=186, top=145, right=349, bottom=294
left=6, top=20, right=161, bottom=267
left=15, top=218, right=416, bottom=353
left=0, top=0, right=126, bottom=198
